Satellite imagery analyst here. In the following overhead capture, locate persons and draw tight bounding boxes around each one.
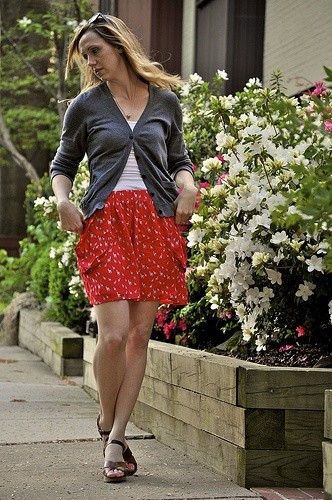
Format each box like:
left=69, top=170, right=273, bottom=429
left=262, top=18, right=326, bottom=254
left=49, top=16, right=198, bottom=482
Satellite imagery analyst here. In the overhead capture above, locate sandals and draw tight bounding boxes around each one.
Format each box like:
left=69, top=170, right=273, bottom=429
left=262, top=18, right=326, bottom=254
left=97, top=413, right=138, bottom=476
left=103, top=436, right=127, bottom=482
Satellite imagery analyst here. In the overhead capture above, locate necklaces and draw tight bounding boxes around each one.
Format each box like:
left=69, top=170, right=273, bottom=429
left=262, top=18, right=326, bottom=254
left=111, top=79, right=138, bottom=119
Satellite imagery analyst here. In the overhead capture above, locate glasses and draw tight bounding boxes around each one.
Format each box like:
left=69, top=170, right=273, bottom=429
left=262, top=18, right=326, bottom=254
left=88, top=12, right=111, bottom=26
